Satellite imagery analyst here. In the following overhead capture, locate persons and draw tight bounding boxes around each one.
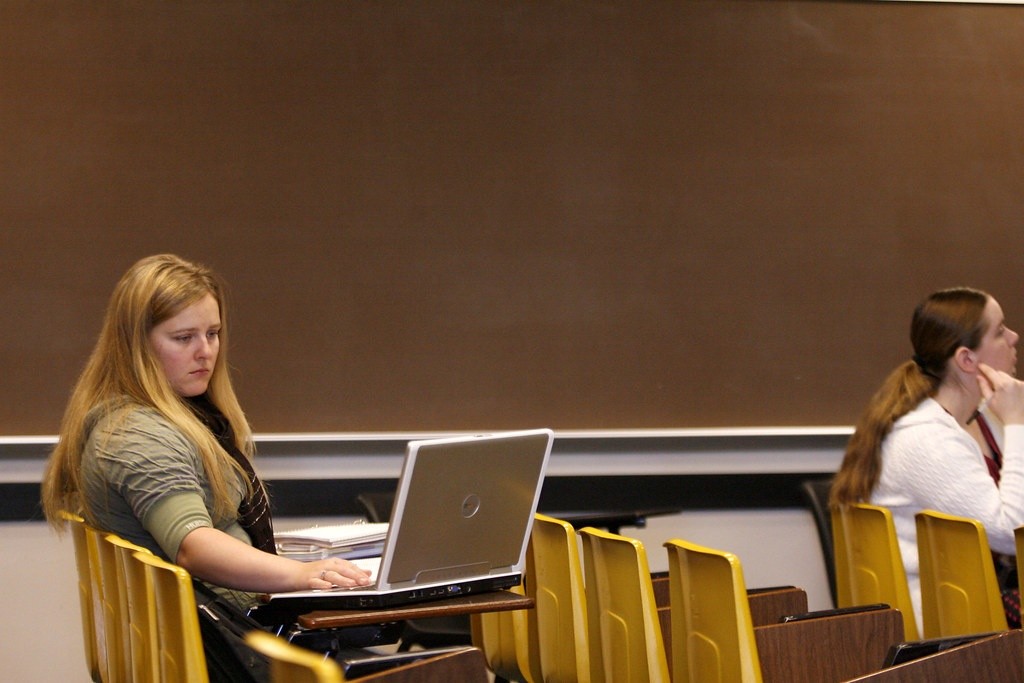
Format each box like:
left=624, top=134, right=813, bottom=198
left=827, top=287, right=1024, bottom=638
left=41, top=253, right=372, bottom=631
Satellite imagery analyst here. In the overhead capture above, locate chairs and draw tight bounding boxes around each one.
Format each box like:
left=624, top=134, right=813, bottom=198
left=56, top=479, right=1024, bottom=683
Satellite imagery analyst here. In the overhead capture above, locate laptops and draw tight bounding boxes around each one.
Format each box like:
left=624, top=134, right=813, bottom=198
left=267, top=427, right=556, bottom=614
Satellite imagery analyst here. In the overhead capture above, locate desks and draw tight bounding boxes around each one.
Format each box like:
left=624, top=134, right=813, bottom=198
left=255, top=592, right=534, bottom=629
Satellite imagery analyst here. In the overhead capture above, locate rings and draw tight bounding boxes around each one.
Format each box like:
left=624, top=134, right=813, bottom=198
left=320, top=572, right=325, bottom=580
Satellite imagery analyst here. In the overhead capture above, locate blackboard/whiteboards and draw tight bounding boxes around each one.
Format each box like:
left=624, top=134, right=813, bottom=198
left=0, top=0, right=1024, bottom=447
